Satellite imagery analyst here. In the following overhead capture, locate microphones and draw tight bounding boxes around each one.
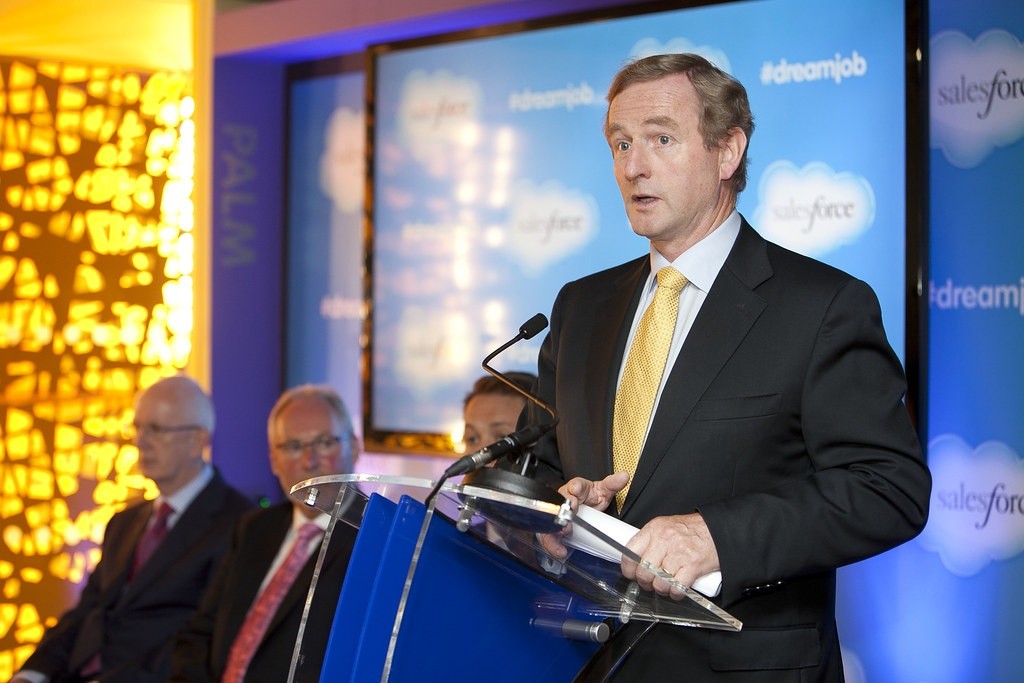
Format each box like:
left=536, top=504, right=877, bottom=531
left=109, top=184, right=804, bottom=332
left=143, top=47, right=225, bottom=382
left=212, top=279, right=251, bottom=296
left=446, top=312, right=560, bottom=475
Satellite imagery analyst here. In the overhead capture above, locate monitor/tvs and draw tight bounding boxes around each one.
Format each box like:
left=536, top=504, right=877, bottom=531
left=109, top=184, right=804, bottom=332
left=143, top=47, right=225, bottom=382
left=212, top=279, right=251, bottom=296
left=369, top=0, right=928, bottom=460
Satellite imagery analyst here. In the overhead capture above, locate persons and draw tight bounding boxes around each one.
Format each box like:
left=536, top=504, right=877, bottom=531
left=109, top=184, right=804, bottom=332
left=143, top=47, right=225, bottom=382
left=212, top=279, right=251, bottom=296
left=9, top=376, right=260, bottom=683
left=492, top=54, right=933, bottom=683
left=167, top=384, right=369, bottom=683
left=461, top=371, right=538, bottom=569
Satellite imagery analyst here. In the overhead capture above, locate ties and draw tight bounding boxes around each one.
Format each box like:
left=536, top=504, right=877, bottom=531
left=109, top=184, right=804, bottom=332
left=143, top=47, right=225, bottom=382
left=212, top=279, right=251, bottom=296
left=81, top=502, right=173, bottom=677
left=612, top=267, right=689, bottom=515
left=221, top=523, right=325, bottom=681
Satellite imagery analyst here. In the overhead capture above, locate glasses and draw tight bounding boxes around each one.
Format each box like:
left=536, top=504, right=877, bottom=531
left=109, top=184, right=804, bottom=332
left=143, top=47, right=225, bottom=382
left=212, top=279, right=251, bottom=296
left=135, top=422, right=206, bottom=436
left=275, top=436, right=355, bottom=457
